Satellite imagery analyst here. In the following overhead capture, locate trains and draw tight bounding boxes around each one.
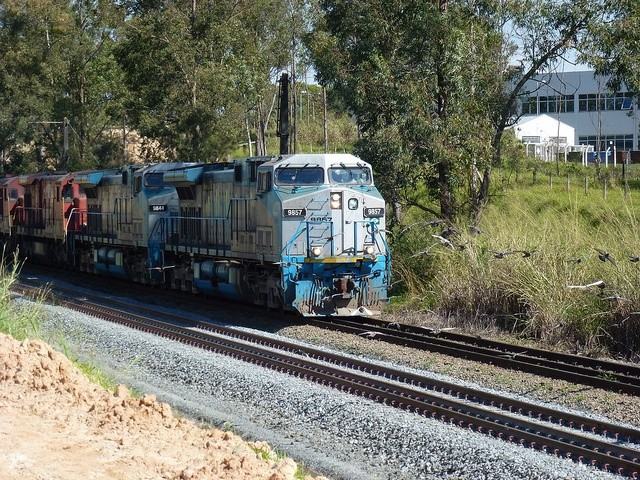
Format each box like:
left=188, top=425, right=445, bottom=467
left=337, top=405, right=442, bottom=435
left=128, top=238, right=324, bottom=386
left=1, top=154, right=391, bottom=317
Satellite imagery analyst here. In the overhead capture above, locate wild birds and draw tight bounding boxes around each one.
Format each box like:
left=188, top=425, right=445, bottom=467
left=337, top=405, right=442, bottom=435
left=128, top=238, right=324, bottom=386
left=374, top=279, right=402, bottom=293
left=439, top=227, right=462, bottom=238
left=318, top=232, right=344, bottom=248
left=431, top=234, right=456, bottom=252
left=594, top=248, right=618, bottom=268
left=398, top=222, right=421, bottom=241
left=420, top=326, right=457, bottom=335
left=413, top=308, right=437, bottom=317
left=148, top=264, right=176, bottom=273
left=320, top=294, right=343, bottom=303
left=387, top=322, right=400, bottom=331
left=488, top=249, right=515, bottom=259
left=359, top=331, right=392, bottom=338
left=407, top=244, right=436, bottom=264
left=372, top=228, right=396, bottom=240
left=420, top=219, right=448, bottom=229
left=412, top=294, right=428, bottom=303
left=628, top=255, right=640, bottom=264
left=272, top=261, right=304, bottom=271
left=213, top=259, right=242, bottom=270
left=513, top=249, right=540, bottom=258
